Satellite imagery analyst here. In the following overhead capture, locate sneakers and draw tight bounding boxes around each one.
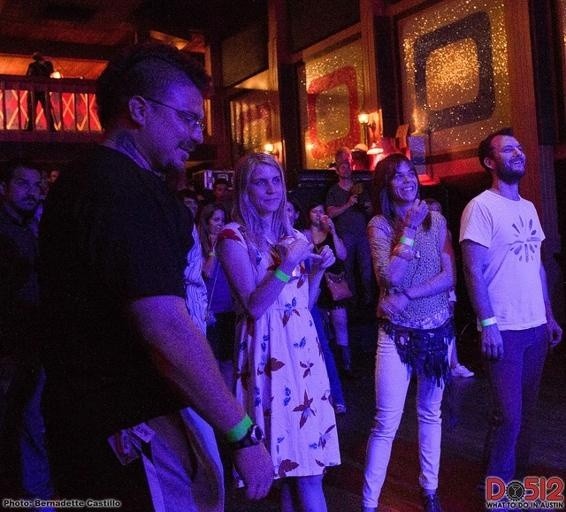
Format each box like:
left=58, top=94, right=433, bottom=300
left=423, top=492, right=442, bottom=512
left=450, top=363, right=474, bottom=378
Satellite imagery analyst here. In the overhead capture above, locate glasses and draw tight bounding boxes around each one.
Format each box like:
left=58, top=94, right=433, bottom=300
left=139, top=92, right=206, bottom=130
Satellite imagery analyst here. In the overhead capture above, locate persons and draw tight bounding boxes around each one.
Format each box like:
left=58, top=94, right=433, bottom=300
left=0, top=46, right=563, bottom=512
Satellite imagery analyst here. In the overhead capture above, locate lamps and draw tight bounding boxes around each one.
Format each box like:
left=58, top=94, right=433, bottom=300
left=357, top=104, right=375, bottom=132
left=264, top=138, right=279, bottom=158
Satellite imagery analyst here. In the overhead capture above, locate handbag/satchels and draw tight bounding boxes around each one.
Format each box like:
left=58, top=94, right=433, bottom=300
left=206, top=311, right=235, bottom=361
left=325, top=271, right=353, bottom=301
